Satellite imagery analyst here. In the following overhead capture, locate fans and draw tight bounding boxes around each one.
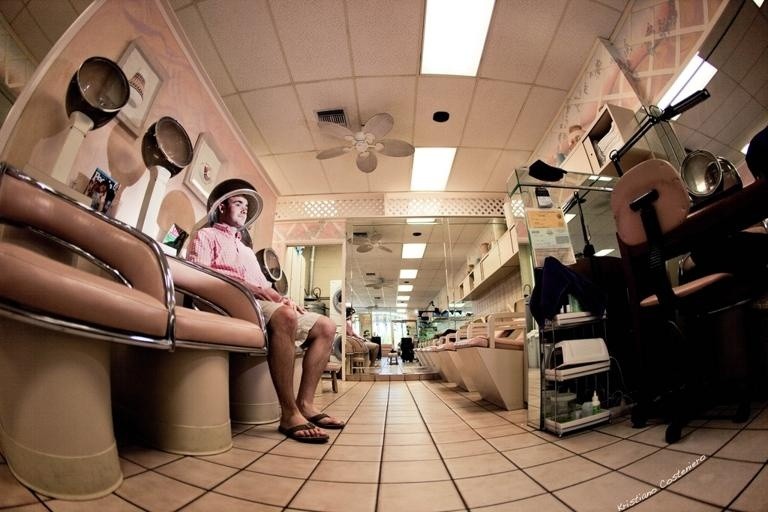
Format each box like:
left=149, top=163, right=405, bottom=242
left=316, top=113, right=415, bottom=173
left=347, top=231, right=393, bottom=253
left=365, top=278, right=395, bottom=290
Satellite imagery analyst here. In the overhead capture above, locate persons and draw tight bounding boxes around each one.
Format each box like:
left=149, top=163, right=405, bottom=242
left=346, top=307, right=380, bottom=367
left=185, top=194, right=346, bottom=443
left=93, top=179, right=109, bottom=193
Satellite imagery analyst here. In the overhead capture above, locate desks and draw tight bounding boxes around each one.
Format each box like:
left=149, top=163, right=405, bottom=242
left=616, top=177, right=767, bottom=401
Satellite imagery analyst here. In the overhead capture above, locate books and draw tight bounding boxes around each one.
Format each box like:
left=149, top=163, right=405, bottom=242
left=161, top=223, right=189, bottom=256
left=83, top=168, right=121, bottom=214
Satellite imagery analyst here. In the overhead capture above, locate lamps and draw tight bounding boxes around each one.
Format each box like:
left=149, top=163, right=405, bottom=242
left=342, top=131, right=383, bottom=159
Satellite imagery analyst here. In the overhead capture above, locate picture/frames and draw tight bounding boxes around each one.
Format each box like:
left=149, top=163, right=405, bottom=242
left=182, top=131, right=230, bottom=207
left=116, top=36, right=169, bottom=138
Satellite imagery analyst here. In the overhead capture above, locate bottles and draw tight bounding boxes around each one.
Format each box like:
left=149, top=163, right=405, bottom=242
left=542, top=389, right=558, bottom=416
left=550, top=391, right=577, bottom=421
left=592, top=390, right=601, bottom=415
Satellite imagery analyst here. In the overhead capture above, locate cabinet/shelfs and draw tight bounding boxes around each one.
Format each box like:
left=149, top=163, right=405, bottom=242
left=507, top=102, right=651, bottom=267
left=459, top=230, right=507, bottom=300
left=540, top=316, right=613, bottom=438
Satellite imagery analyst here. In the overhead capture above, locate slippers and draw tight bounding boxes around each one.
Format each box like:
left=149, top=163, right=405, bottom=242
left=273, top=417, right=329, bottom=446
left=302, top=407, right=346, bottom=430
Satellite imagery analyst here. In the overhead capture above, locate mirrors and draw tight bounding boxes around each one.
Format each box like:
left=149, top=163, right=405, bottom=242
left=344, top=215, right=507, bottom=376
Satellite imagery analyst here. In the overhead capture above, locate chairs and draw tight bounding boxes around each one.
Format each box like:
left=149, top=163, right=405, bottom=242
left=610, top=158, right=751, bottom=444
left=0, top=163, right=306, bottom=501
left=426, top=299, right=525, bottom=411
left=346, top=336, right=369, bottom=374
left=413, top=329, right=446, bottom=373
left=381, top=344, right=393, bottom=357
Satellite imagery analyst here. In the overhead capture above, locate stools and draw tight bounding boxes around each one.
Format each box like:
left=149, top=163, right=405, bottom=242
left=388, top=352, right=398, bottom=365
left=321, top=362, right=342, bottom=393
